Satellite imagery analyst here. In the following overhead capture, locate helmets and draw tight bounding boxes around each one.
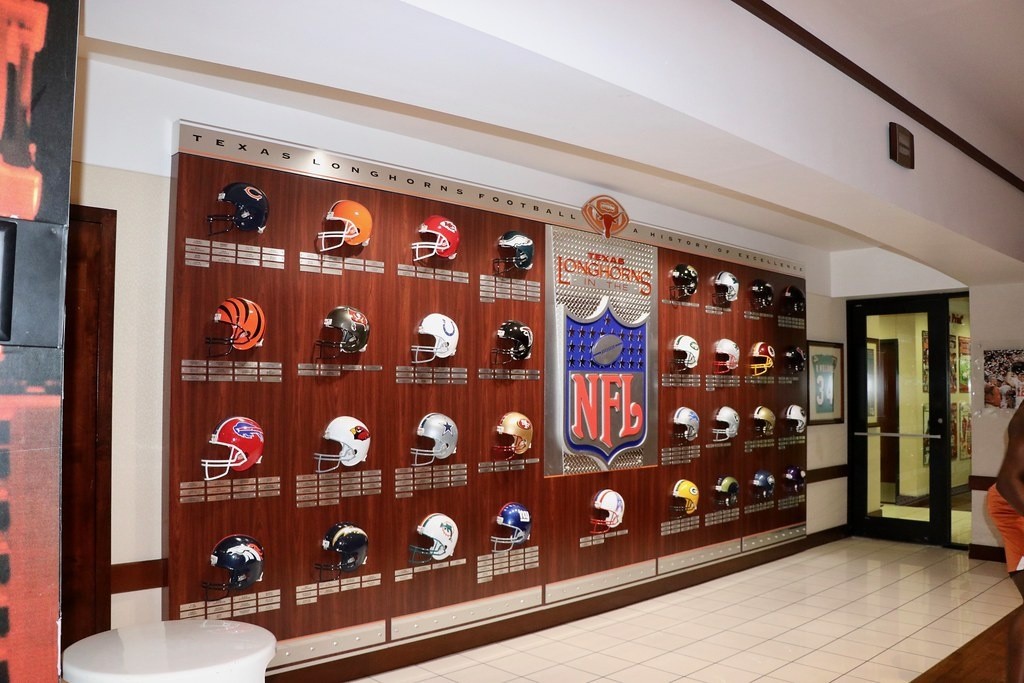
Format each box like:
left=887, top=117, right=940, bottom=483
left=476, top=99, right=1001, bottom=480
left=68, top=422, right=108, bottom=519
left=667, top=264, right=808, bottom=519
left=192, top=183, right=532, bottom=592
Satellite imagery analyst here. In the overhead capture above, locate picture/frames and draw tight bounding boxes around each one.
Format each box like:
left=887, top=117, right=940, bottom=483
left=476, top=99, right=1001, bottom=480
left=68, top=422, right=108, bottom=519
left=867, top=338, right=881, bottom=427
left=806, top=340, right=845, bottom=425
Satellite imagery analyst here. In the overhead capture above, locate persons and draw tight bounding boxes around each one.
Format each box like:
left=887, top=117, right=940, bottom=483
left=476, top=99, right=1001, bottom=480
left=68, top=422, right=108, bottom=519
left=988, top=399, right=1024, bottom=683
left=983, top=350, right=1024, bottom=409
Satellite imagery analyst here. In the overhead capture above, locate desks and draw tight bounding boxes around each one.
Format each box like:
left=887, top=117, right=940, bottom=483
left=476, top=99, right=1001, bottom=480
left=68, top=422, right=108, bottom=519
left=62, top=618, right=277, bottom=683
